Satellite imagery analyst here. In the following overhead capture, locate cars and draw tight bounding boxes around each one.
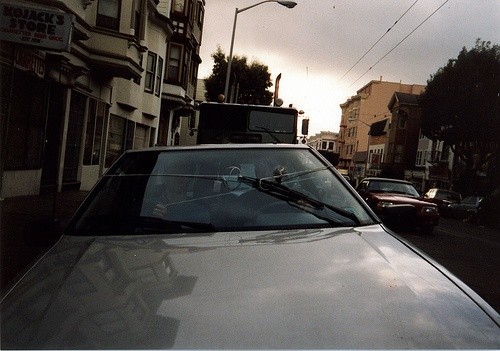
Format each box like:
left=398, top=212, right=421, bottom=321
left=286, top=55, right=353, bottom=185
left=422, top=188, right=500, bottom=224
left=0, top=145, right=500, bottom=350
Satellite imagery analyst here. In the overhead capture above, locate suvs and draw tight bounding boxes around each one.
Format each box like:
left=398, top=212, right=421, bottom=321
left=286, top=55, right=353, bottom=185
left=355, top=176, right=440, bottom=233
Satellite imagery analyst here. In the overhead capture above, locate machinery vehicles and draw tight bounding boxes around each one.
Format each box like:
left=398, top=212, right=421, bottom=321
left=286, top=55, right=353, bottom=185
left=194, top=73, right=309, bottom=146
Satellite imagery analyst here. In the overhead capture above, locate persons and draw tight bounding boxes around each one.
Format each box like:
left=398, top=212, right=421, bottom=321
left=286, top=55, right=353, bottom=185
left=140, top=156, right=211, bottom=224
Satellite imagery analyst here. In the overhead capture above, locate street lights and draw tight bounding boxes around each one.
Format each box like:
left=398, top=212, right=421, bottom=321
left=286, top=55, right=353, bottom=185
left=348, top=118, right=372, bottom=177
left=222, top=0, right=298, bottom=103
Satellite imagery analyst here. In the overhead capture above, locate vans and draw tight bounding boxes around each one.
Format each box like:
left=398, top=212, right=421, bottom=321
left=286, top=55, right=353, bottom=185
left=335, top=169, right=354, bottom=188
